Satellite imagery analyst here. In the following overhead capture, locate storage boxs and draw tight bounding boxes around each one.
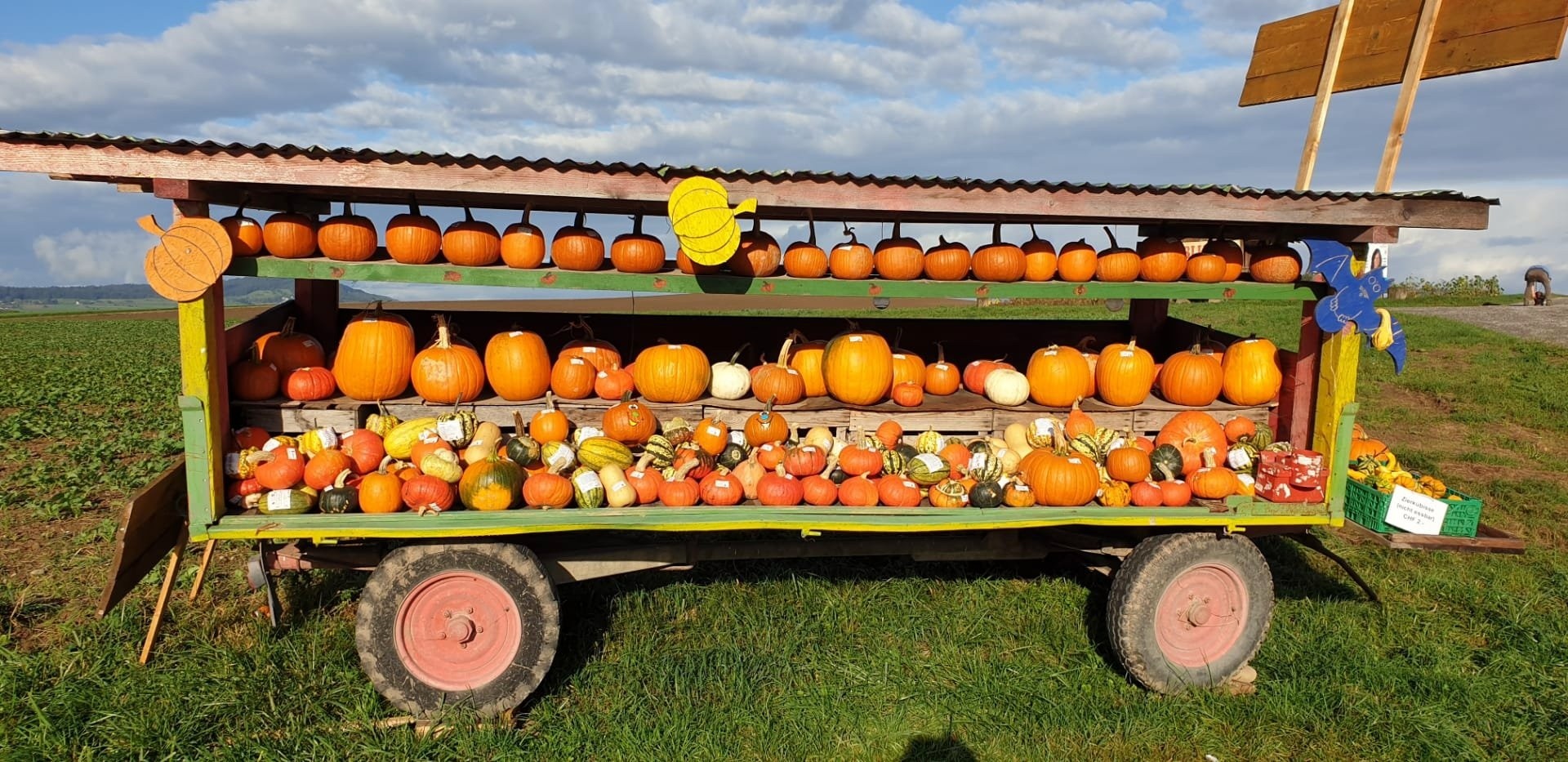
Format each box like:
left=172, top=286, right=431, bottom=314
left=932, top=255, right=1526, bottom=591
left=231, top=398, right=1270, bottom=446
left=1344, top=457, right=1483, bottom=533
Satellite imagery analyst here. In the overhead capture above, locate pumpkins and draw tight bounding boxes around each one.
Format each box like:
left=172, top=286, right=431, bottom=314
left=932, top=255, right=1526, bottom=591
left=1348, top=424, right=1463, bottom=501
left=219, top=203, right=1304, bottom=518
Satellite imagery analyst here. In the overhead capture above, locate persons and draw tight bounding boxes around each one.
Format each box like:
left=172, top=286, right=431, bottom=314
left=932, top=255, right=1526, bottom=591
left=1371, top=248, right=1382, bottom=273
